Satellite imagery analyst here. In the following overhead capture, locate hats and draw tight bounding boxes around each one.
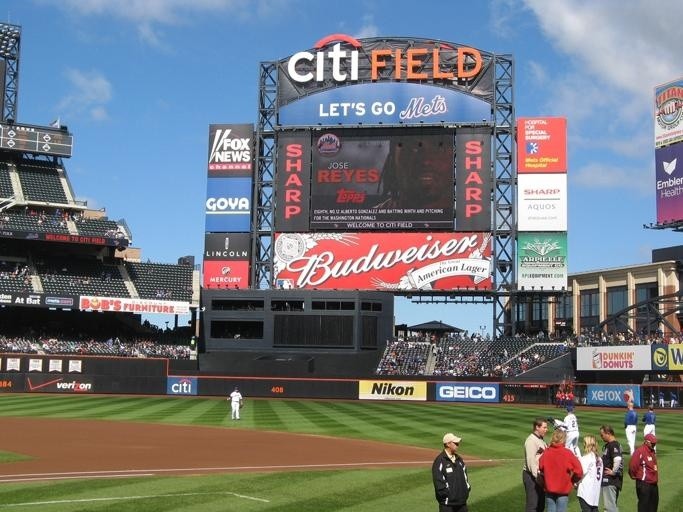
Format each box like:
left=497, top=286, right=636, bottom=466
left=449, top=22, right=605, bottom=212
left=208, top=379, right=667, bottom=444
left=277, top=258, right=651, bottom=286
left=443, top=433, right=462, bottom=444
left=644, top=434, right=657, bottom=444
left=567, top=407, right=573, bottom=411
left=235, top=386, right=239, bottom=389
left=547, top=416, right=553, bottom=420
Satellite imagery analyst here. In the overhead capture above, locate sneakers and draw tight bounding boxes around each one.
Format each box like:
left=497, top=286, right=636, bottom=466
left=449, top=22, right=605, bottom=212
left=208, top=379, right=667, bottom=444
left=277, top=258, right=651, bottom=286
left=232, top=416, right=240, bottom=420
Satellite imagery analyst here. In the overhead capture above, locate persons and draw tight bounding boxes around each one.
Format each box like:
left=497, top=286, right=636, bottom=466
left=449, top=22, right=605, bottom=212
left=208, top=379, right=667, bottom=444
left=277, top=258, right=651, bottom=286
left=225, top=385, right=244, bottom=421
left=430, top=432, right=475, bottom=511
left=574, top=434, right=605, bottom=512
left=640, top=402, right=657, bottom=454
left=553, top=404, right=581, bottom=460
left=599, top=424, right=623, bottom=512
left=519, top=416, right=546, bottom=512
left=538, top=428, right=583, bottom=512
left=623, top=400, right=639, bottom=456
left=625, top=433, right=660, bottom=512
left=373, top=318, right=682, bottom=414
left=0, top=206, right=193, bottom=359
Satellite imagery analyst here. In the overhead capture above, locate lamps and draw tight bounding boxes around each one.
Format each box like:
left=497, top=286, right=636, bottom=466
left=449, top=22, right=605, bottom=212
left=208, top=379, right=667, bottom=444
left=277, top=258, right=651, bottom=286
left=408, top=283, right=567, bottom=305
left=640, top=218, right=682, bottom=234
left=203, top=279, right=402, bottom=299
left=274, top=115, right=491, bottom=130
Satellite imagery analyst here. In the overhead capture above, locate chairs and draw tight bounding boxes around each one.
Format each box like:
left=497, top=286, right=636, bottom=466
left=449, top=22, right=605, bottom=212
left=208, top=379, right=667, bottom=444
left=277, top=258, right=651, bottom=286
left=372, top=331, right=577, bottom=379
left=0, top=158, right=193, bottom=360
